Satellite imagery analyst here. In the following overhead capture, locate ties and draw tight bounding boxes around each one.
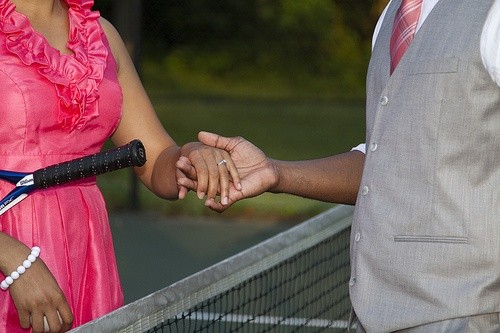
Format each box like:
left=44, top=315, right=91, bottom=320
left=390, top=0, right=423, bottom=77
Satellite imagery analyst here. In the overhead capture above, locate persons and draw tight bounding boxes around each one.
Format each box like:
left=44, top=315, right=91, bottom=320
left=177, top=0, right=500, bottom=333
left=0, top=1, right=242, bottom=333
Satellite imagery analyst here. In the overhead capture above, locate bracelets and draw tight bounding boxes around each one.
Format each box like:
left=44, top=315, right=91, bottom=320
left=0, top=246, right=41, bottom=293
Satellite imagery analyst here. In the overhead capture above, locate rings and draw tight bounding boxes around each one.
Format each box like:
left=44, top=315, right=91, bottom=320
left=217, top=160, right=226, bottom=166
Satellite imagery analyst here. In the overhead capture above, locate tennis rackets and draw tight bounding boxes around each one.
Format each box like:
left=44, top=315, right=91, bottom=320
left=0, top=139, right=147, bottom=216
